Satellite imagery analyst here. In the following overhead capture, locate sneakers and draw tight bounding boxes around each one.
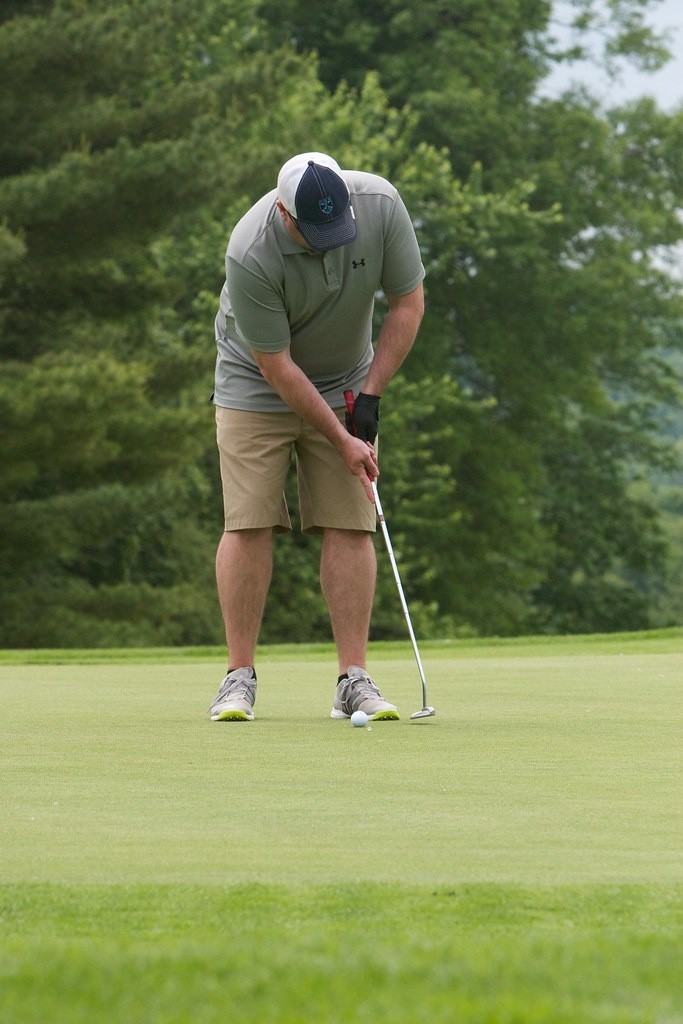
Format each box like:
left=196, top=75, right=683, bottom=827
left=207, top=667, right=258, bottom=722
left=331, top=664, right=400, bottom=721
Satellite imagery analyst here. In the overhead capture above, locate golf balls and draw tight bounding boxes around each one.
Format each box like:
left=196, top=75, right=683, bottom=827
left=351, top=710, right=369, bottom=726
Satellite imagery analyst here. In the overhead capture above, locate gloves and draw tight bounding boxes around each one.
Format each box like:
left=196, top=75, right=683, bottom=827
left=345, top=391, right=381, bottom=446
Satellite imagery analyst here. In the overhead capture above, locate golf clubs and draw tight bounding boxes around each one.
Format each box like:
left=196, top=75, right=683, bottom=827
left=343, top=389, right=437, bottom=720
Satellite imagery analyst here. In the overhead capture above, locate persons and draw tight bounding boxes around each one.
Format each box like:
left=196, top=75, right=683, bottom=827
left=208, top=153, right=425, bottom=721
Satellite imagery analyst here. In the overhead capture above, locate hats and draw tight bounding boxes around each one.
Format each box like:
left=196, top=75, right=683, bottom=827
left=277, top=152, right=358, bottom=251
